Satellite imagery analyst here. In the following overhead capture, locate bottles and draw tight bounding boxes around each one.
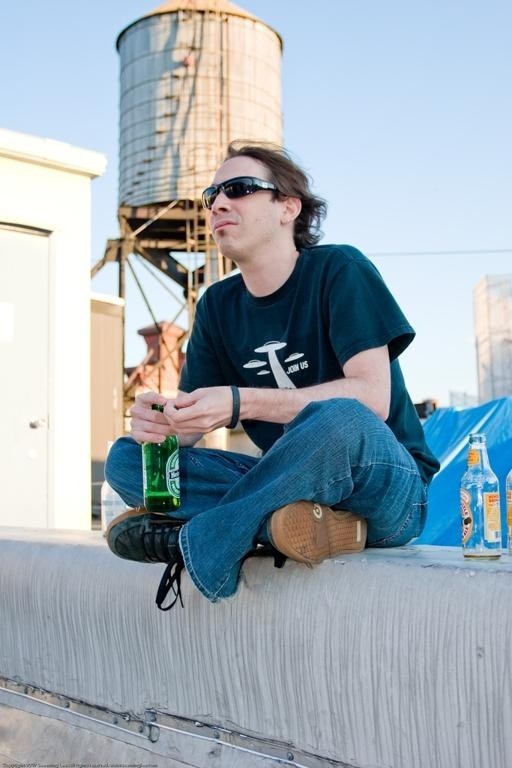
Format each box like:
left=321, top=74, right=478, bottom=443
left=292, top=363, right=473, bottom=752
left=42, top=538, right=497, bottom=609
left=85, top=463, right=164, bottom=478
left=98, top=440, right=134, bottom=540
left=138, top=401, right=181, bottom=514
left=460, top=428, right=505, bottom=563
left=505, top=468, right=512, bottom=557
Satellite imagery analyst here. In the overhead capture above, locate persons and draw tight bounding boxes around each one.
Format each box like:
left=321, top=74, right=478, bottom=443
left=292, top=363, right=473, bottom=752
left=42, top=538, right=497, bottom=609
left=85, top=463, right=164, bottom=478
left=104, top=137, right=440, bottom=610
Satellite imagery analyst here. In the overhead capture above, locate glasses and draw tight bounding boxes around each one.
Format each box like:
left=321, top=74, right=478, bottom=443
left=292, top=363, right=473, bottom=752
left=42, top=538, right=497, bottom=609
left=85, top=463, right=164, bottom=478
left=202, top=176, right=278, bottom=209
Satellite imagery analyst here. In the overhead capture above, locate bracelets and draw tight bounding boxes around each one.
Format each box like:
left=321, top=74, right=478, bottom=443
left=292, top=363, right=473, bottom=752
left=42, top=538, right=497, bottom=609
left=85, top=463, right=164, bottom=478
left=225, top=386, right=241, bottom=429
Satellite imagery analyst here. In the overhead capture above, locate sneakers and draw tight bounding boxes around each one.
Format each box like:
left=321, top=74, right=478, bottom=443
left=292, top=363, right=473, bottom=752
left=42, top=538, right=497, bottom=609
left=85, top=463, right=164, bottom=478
left=266, top=500, right=367, bottom=564
left=106, top=509, right=189, bottom=563
